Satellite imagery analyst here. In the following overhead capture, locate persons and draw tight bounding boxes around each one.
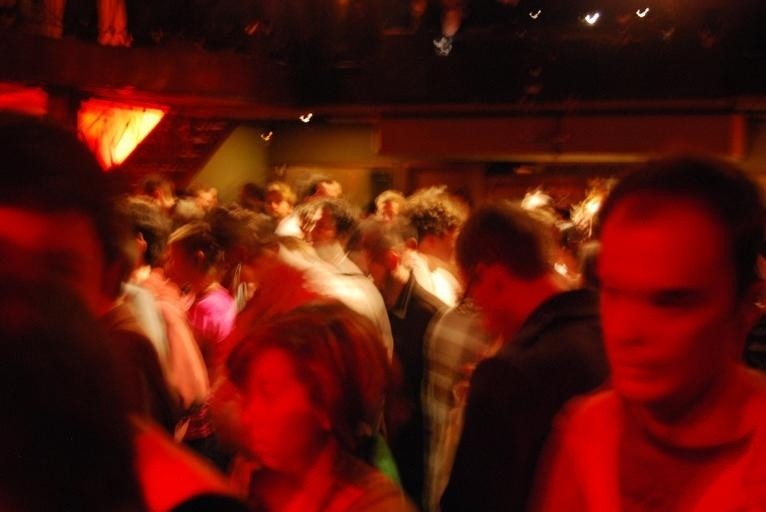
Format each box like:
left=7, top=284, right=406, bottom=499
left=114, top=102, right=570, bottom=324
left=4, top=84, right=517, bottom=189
left=0, top=103, right=766, bottom=512
